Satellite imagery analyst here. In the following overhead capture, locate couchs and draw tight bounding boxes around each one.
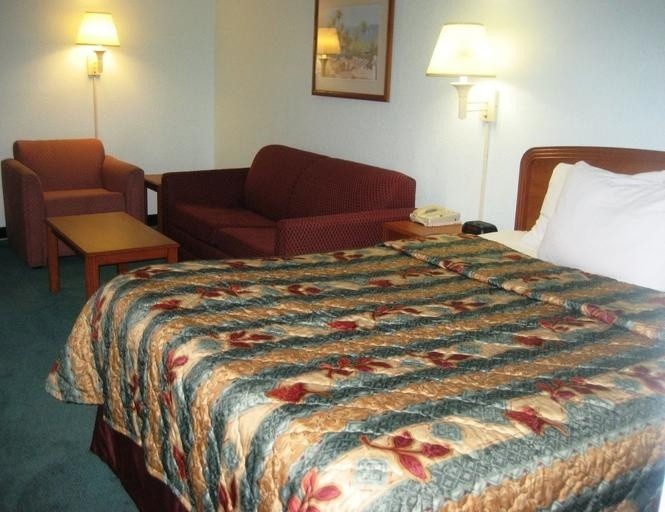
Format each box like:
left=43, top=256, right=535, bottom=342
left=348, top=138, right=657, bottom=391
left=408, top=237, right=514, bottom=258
left=156, top=144, right=418, bottom=263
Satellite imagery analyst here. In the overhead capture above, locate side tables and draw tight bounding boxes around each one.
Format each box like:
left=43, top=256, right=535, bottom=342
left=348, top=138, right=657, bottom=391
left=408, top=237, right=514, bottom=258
left=144, top=174, right=161, bottom=224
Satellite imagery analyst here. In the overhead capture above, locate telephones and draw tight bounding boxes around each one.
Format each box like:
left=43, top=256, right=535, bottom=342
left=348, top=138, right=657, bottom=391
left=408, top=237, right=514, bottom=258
left=409, top=205, right=461, bottom=226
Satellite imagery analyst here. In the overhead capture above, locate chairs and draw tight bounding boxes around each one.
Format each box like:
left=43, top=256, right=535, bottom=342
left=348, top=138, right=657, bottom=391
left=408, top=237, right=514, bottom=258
left=0, top=137, right=147, bottom=268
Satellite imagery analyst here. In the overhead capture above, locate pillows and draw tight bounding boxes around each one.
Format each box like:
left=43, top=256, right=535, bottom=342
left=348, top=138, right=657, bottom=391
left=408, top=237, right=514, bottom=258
left=518, top=158, right=665, bottom=295
left=537, top=160, right=664, bottom=294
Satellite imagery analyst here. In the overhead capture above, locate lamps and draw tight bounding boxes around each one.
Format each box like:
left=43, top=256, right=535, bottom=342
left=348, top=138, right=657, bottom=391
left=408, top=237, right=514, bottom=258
left=316, top=27, right=342, bottom=77
left=74, top=11, right=121, bottom=76
left=425, top=22, right=500, bottom=123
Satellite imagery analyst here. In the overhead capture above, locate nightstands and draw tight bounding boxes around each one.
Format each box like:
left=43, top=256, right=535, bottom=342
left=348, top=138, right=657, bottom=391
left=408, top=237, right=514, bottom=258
left=380, top=221, right=464, bottom=243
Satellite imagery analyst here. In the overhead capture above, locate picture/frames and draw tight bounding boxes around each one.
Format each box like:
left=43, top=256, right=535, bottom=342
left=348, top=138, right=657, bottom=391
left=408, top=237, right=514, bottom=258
left=311, top=0, right=395, bottom=102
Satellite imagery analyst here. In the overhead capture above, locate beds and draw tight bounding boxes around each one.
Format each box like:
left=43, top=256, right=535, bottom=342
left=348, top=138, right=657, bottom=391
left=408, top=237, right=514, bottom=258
left=44, top=145, right=665, bottom=512
